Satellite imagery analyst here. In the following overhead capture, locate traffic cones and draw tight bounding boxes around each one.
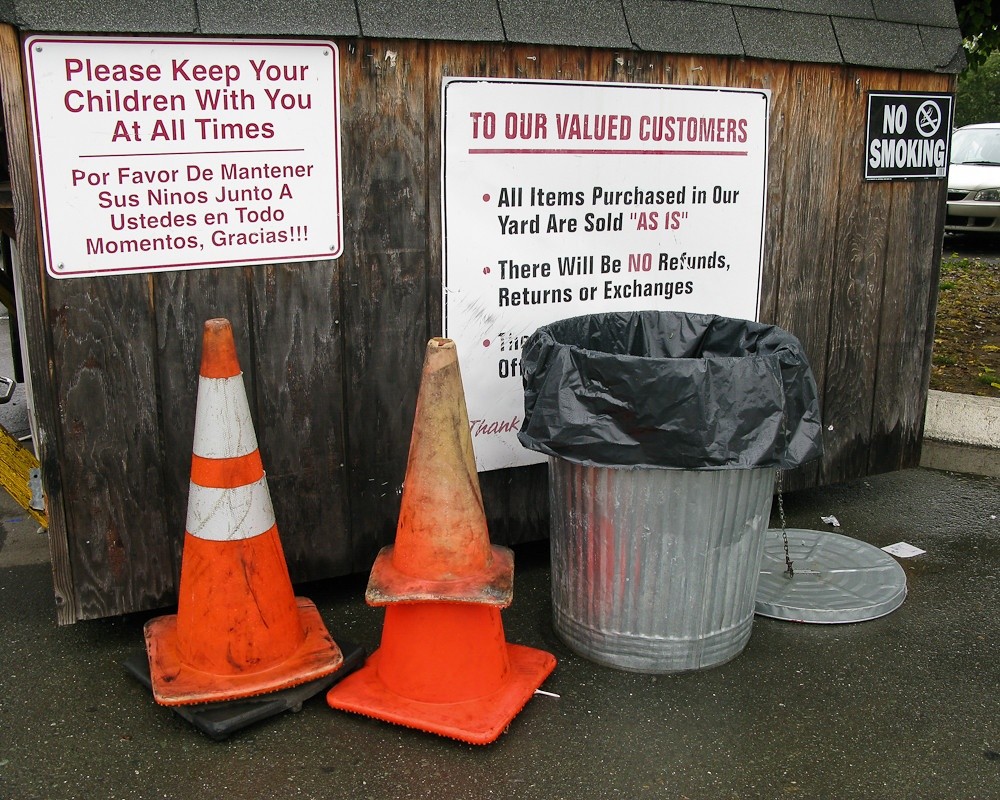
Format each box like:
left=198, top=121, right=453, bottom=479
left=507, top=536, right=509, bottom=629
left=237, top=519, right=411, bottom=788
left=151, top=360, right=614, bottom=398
left=119, top=314, right=363, bottom=744
left=319, top=581, right=562, bottom=745
left=366, top=339, right=516, bottom=609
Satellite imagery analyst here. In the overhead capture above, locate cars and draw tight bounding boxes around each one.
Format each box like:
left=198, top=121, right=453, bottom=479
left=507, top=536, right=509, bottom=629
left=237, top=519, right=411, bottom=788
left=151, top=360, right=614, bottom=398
left=944, top=123, right=999, bottom=237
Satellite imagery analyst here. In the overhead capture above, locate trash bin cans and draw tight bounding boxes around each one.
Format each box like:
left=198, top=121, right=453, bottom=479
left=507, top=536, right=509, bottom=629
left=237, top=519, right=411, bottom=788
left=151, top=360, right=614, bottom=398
left=515, top=307, right=824, bottom=677
